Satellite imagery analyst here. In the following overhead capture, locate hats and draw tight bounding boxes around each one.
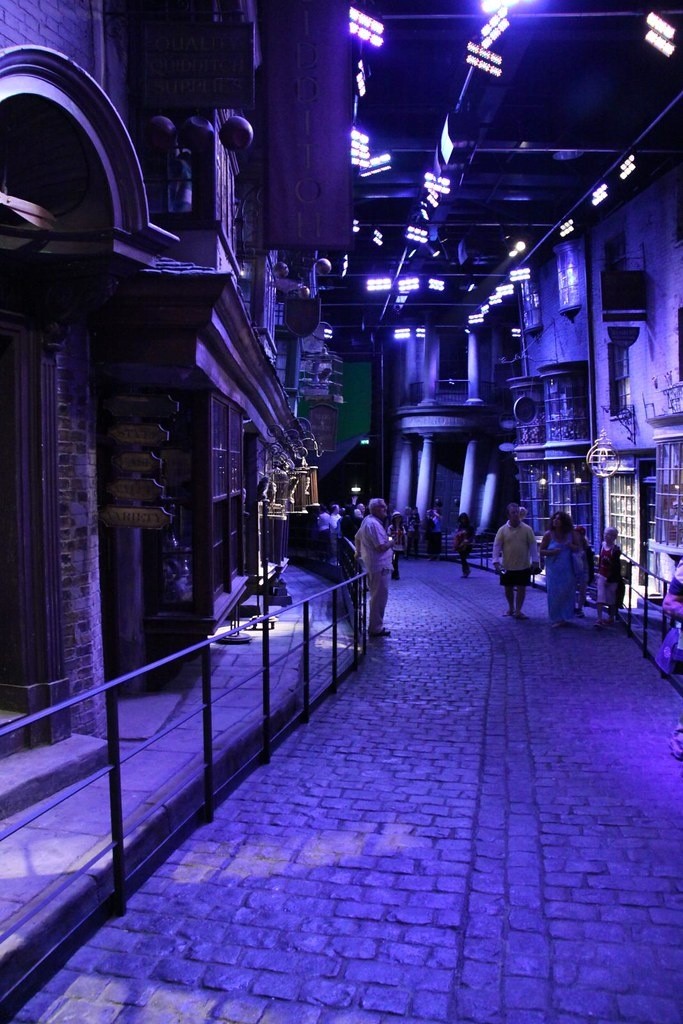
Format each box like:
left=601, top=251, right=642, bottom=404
left=391, top=512, right=404, bottom=523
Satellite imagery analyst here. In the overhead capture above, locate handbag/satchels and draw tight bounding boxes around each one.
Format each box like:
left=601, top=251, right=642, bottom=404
left=572, top=551, right=584, bottom=579
left=655, top=616, right=679, bottom=675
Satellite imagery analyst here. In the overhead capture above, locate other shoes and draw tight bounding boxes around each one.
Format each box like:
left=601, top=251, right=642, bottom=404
left=502, top=608, right=514, bottom=616
left=575, top=608, right=585, bottom=617
left=513, top=609, right=527, bottom=619
left=602, top=619, right=614, bottom=625
left=550, top=622, right=565, bottom=627
left=593, top=618, right=602, bottom=626
left=668, top=729, right=683, bottom=759
left=368, top=628, right=391, bottom=636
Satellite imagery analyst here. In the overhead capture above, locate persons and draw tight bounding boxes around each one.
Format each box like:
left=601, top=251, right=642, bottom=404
left=593, top=527, right=621, bottom=627
left=426, top=508, right=444, bottom=561
left=539, top=511, right=595, bottom=626
left=354, top=498, right=396, bottom=635
left=662, top=554, right=683, bottom=763
left=319, top=503, right=407, bottom=580
left=457, top=513, right=473, bottom=578
left=492, top=503, right=538, bottom=619
left=405, top=507, right=424, bottom=561
